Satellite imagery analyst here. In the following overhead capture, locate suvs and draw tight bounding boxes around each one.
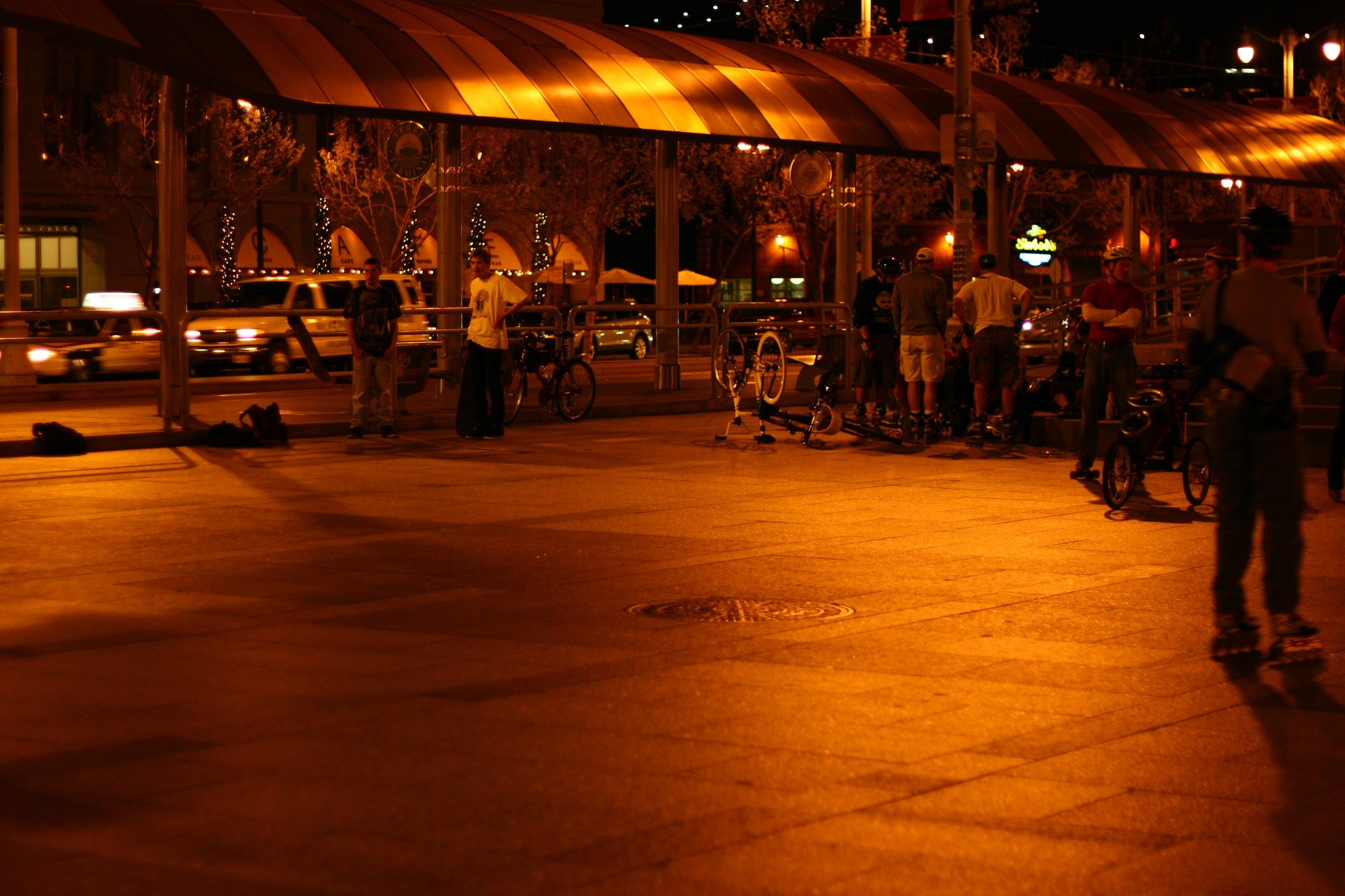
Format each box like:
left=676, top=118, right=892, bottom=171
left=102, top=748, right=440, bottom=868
left=181, top=271, right=434, bottom=375
left=535, top=302, right=653, bottom=360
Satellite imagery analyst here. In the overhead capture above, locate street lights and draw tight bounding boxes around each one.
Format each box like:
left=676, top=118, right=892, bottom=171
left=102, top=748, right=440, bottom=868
left=775, top=234, right=789, bottom=303
left=738, top=143, right=771, bottom=299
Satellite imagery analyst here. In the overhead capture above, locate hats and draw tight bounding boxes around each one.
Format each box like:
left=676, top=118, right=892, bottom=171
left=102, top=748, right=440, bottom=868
left=916, top=247, right=935, bottom=261
left=978, top=253, right=997, bottom=270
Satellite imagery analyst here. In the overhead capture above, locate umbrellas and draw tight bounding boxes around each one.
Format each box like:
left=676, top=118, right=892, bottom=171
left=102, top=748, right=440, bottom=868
left=533, top=266, right=728, bottom=324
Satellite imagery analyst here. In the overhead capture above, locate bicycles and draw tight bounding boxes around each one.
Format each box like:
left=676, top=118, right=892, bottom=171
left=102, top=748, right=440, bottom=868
left=711, top=328, right=788, bottom=445
left=755, top=381, right=903, bottom=448
left=499, top=329, right=597, bottom=427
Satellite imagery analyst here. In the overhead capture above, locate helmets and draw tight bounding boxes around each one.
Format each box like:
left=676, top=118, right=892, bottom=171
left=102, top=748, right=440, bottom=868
left=1103, top=246, right=1133, bottom=262
left=1205, top=246, right=1240, bottom=271
left=1244, top=205, right=1294, bottom=245
left=877, top=258, right=902, bottom=273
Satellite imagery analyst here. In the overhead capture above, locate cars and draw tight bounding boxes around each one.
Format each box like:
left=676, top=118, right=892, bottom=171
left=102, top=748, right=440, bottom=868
left=720, top=301, right=850, bottom=350
left=18, top=292, right=167, bottom=380
left=1023, top=320, right=1060, bottom=343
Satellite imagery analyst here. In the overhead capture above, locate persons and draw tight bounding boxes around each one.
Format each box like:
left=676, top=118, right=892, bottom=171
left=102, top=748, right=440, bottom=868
left=851, top=247, right=1345, bottom=505
left=344, top=258, right=402, bottom=439
left=1195, top=204, right=1322, bottom=665
left=465, top=247, right=529, bottom=439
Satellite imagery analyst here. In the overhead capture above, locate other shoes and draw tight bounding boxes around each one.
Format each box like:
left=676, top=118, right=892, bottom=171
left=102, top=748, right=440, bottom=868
left=483, top=431, right=504, bottom=439
left=346, top=428, right=364, bottom=438
left=382, top=425, right=398, bottom=438
left=465, top=433, right=485, bottom=438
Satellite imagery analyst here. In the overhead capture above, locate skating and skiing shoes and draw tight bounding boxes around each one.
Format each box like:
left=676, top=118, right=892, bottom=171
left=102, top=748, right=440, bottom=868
left=1001, top=413, right=1020, bottom=446
left=1069, top=453, right=1101, bottom=480
left=986, top=412, right=1004, bottom=436
left=1120, top=459, right=1146, bottom=480
left=895, top=403, right=953, bottom=445
left=1270, top=609, right=1323, bottom=663
left=1210, top=610, right=1260, bottom=658
left=964, top=413, right=985, bottom=446
left=871, top=406, right=896, bottom=436
left=854, top=407, right=871, bottom=430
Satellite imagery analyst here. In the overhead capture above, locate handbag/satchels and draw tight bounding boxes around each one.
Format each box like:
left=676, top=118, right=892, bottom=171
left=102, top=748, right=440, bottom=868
left=32, top=421, right=88, bottom=455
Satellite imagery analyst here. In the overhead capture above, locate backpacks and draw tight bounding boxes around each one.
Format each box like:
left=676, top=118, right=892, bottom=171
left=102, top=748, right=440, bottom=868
left=240, top=402, right=288, bottom=444
